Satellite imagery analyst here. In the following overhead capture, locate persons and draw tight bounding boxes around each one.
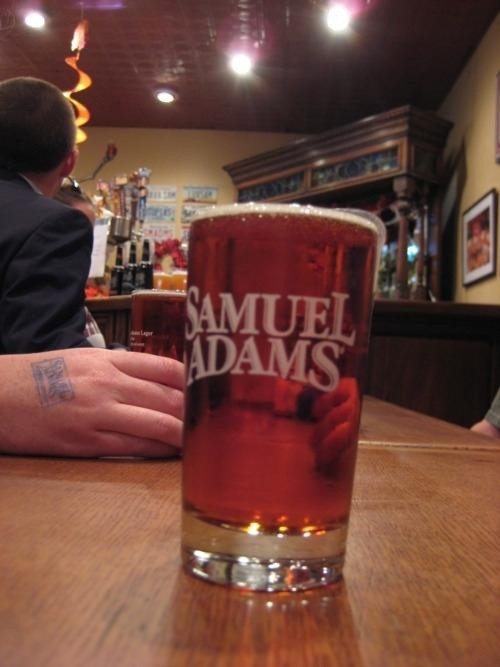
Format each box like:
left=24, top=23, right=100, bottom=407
left=470, top=384, right=500, bottom=439
left=0, top=78, right=95, bottom=356
left=50, top=183, right=106, bottom=349
left=0, top=347, right=187, bottom=461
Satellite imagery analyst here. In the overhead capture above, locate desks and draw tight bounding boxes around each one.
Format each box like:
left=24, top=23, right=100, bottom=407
left=0, top=393, right=500, bottom=667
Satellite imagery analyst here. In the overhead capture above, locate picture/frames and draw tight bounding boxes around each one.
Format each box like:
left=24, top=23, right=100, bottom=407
left=459, top=187, right=497, bottom=288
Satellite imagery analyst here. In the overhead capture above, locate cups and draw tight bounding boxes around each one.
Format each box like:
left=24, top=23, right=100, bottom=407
left=129, top=205, right=387, bottom=594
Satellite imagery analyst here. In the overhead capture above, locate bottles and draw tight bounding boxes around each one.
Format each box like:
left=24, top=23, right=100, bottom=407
left=109, top=235, right=137, bottom=296
